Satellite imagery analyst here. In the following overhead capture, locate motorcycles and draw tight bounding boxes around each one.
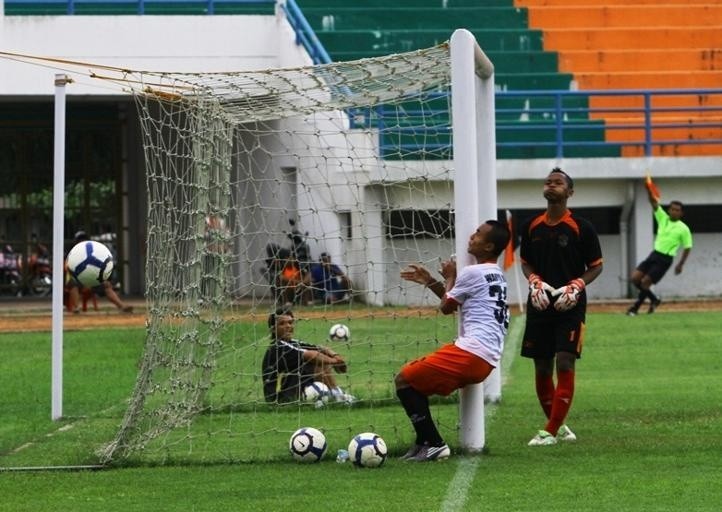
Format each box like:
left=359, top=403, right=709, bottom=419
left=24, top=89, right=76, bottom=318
left=25, top=229, right=52, bottom=303
left=263, top=217, right=316, bottom=306
left=0, top=231, right=26, bottom=300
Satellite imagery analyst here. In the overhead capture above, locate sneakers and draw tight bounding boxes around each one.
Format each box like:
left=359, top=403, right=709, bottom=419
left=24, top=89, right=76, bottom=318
left=330, top=385, right=356, bottom=403
left=557, top=424, right=576, bottom=442
left=528, top=430, right=558, bottom=446
left=399, top=445, right=422, bottom=460
left=627, top=307, right=638, bottom=315
left=648, top=298, right=661, bottom=313
left=405, top=441, right=450, bottom=462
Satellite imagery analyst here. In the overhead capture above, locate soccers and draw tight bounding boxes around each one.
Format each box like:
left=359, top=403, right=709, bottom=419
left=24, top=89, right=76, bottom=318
left=348, top=433, right=388, bottom=468
left=304, top=381, right=331, bottom=403
left=290, top=428, right=327, bottom=463
left=330, top=324, right=349, bottom=341
left=66, top=241, right=113, bottom=288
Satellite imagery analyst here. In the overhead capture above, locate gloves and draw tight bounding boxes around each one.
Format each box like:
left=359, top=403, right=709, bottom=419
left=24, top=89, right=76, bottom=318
left=550, top=277, right=586, bottom=314
left=332, top=355, right=346, bottom=374
left=527, top=272, right=555, bottom=313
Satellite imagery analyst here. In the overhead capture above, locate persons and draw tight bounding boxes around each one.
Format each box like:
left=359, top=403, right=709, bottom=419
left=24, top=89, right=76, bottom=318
left=260, top=308, right=359, bottom=407
left=626, top=179, right=696, bottom=315
left=518, top=168, right=604, bottom=446
left=392, top=219, right=513, bottom=462
left=63, top=230, right=137, bottom=315
left=294, top=253, right=355, bottom=305
